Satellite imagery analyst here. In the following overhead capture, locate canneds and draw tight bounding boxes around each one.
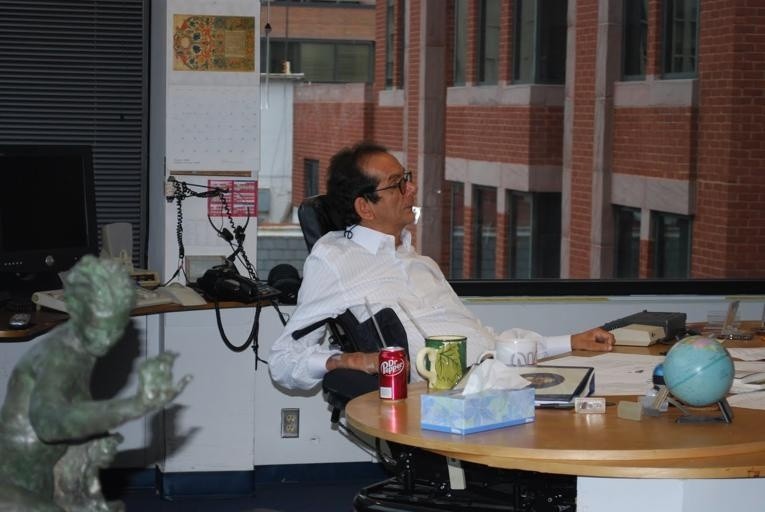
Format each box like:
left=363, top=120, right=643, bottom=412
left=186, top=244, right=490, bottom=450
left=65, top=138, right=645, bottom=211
left=379, top=345, right=407, bottom=403
left=380, top=403, right=408, bottom=435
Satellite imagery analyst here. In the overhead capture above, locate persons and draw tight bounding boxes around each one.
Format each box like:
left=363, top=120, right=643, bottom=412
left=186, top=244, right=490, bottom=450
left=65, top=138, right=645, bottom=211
left=268, top=142, right=615, bottom=488
left=0, top=250, right=194, bottom=512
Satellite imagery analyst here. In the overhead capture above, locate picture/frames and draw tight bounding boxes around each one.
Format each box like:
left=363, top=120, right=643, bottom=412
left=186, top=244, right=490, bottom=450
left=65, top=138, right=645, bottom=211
left=451, top=362, right=594, bottom=403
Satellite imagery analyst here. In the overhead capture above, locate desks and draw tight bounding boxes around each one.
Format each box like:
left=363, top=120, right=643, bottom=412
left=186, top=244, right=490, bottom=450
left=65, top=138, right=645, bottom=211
left=342, top=320, right=765, bottom=512
left=0, top=284, right=279, bottom=498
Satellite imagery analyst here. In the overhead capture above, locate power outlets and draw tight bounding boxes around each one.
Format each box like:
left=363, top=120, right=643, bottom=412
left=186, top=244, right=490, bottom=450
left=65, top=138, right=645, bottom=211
left=281, top=408, right=299, bottom=438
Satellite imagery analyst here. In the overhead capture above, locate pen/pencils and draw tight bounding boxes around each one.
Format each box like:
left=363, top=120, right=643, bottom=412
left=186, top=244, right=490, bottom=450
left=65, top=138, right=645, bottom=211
left=541, top=400, right=616, bottom=410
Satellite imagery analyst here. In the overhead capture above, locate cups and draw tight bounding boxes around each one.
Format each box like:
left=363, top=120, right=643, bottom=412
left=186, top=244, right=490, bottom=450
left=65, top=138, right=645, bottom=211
left=413, top=334, right=469, bottom=391
left=475, top=336, right=538, bottom=367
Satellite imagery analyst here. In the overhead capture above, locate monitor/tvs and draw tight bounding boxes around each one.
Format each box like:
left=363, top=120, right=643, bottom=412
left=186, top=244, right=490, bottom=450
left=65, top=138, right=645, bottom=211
left=0, top=144, right=99, bottom=311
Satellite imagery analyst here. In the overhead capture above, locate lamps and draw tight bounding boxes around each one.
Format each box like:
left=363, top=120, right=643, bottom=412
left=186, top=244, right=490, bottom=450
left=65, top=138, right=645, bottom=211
left=164, top=175, right=261, bottom=282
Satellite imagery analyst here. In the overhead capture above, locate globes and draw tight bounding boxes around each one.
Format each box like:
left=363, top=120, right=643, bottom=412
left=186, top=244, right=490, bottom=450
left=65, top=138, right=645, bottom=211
left=662, top=335, right=735, bottom=425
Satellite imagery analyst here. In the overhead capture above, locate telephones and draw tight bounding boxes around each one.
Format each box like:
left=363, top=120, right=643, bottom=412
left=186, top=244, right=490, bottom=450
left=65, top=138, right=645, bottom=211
left=196, top=268, right=281, bottom=302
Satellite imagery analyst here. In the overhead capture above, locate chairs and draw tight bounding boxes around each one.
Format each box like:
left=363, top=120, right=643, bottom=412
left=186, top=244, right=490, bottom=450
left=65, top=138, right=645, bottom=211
left=291, top=193, right=576, bottom=510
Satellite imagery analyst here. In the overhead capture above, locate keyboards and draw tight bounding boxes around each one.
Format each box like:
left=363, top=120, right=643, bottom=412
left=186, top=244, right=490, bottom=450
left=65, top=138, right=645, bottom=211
left=32, top=283, right=173, bottom=314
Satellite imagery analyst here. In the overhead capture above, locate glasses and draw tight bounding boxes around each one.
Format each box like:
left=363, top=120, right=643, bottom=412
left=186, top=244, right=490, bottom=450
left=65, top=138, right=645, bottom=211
left=362, top=170, right=413, bottom=195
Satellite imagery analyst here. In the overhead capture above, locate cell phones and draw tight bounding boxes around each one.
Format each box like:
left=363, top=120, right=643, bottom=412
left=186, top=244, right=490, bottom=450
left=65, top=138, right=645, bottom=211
left=9, top=312, right=32, bottom=328
